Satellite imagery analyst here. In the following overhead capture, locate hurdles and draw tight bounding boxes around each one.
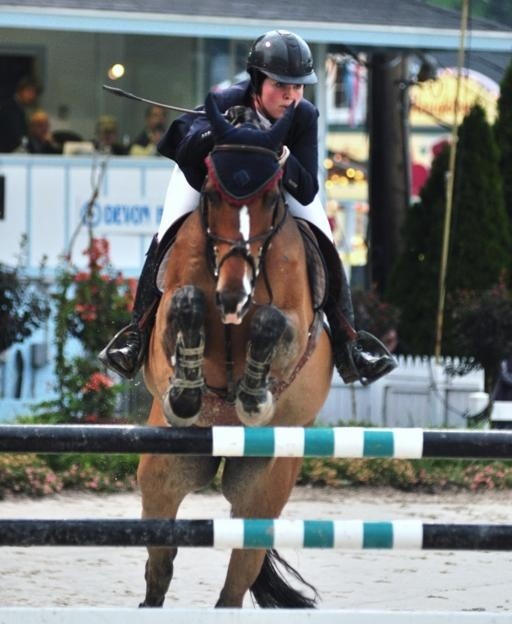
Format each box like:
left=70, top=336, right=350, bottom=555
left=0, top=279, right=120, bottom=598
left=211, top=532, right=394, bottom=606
left=1, top=425, right=511, bottom=621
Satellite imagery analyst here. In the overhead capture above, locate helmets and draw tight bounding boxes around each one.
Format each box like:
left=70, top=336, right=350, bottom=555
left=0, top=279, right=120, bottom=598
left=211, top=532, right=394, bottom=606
left=245, top=29, right=318, bottom=86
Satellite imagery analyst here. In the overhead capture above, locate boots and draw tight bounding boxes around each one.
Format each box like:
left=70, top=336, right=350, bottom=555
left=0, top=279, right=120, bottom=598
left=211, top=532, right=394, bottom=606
left=107, top=233, right=163, bottom=374
left=323, top=267, right=391, bottom=384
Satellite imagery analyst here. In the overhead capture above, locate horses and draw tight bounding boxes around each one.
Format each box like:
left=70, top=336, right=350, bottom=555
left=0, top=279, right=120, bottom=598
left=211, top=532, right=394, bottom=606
left=137, top=91, right=334, bottom=609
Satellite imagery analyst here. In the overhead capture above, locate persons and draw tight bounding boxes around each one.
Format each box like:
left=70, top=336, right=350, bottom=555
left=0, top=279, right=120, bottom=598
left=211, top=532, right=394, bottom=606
left=113, top=29, right=396, bottom=382
left=2, top=80, right=170, bottom=155
